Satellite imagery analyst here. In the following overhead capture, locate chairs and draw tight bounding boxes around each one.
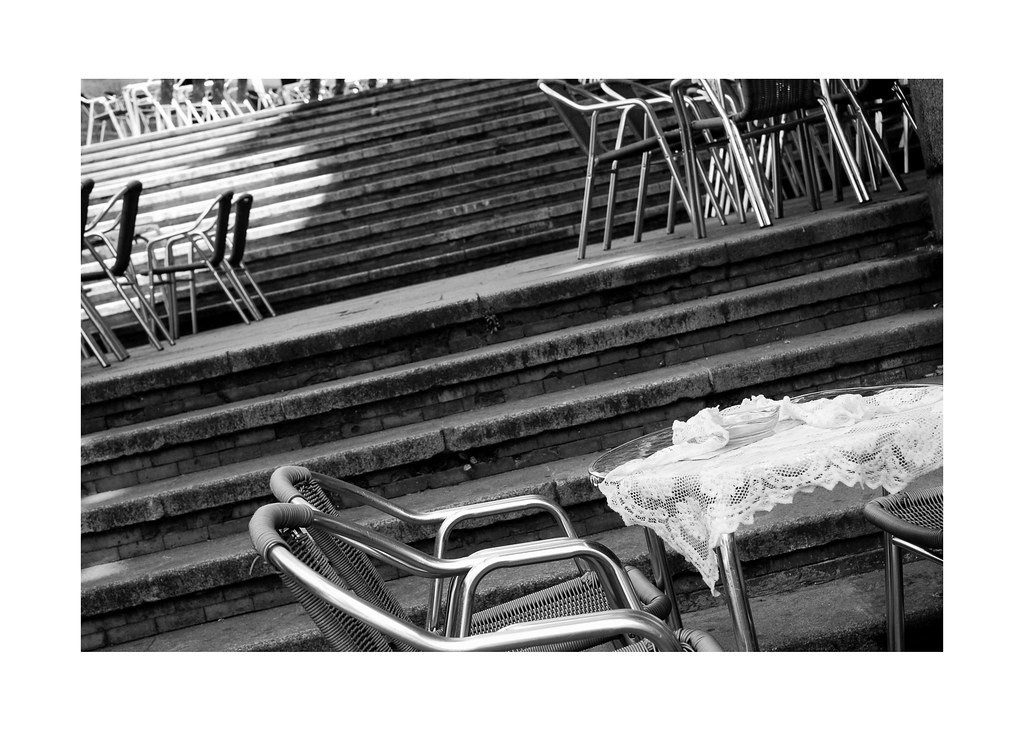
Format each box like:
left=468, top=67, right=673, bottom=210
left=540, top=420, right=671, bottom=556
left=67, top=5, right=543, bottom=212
left=863, top=486, right=943, bottom=652
left=535, top=79, right=917, bottom=260
left=251, top=465, right=722, bottom=652
left=81, top=79, right=327, bottom=145
left=81, top=179, right=272, bottom=368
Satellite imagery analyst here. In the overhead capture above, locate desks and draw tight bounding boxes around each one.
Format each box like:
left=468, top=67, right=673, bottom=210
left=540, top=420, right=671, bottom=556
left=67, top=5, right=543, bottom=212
left=589, top=386, right=943, bottom=652
